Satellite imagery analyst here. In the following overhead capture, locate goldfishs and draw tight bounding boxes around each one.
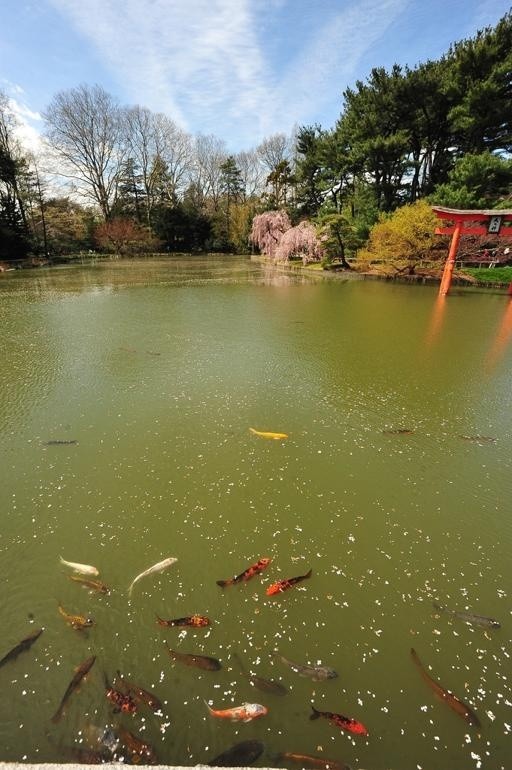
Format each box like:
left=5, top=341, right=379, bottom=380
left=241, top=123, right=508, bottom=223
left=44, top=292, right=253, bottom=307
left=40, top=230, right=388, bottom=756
left=45, top=649, right=371, bottom=764
